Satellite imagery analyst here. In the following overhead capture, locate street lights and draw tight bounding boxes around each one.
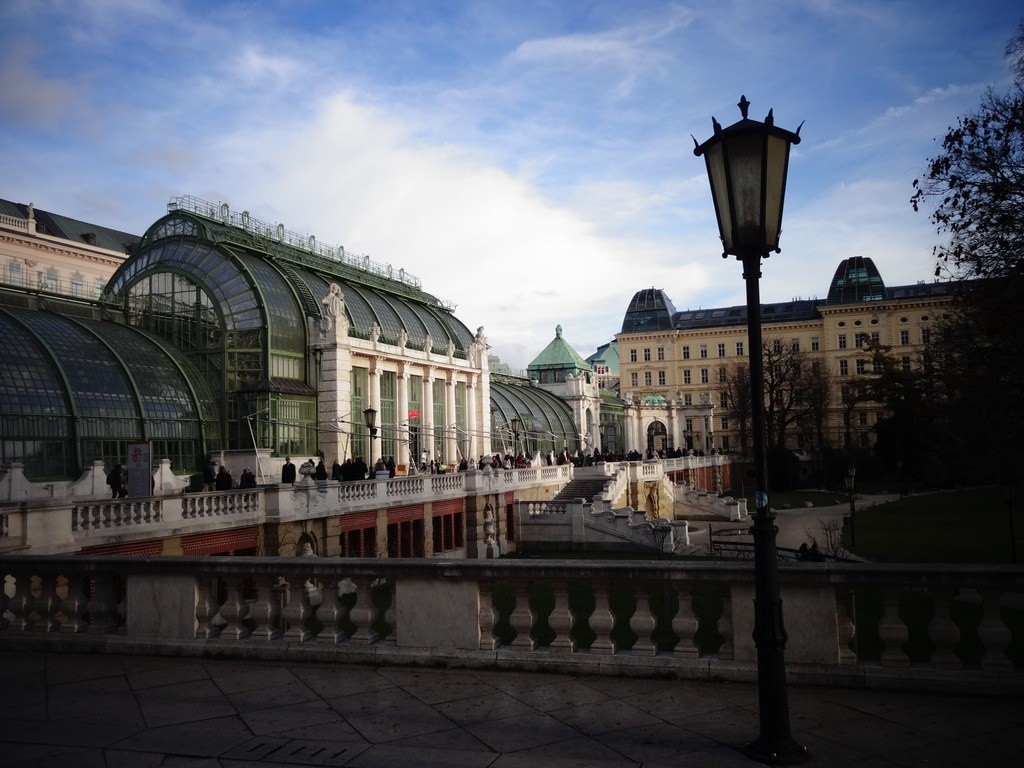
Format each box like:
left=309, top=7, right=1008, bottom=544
left=690, top=95, right=811, bottom=768
left=841, top=461, right=857, bottom=545
left=363, top=405, right=378, bottom=479
left=511, top=416, right=521, bottom=469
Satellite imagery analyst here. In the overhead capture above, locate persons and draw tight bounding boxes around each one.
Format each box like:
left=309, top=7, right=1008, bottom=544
left=798, top=543, right=818, bottom=560
left=201, top=446, right=715, bottom=490
left=110, top=464, right=155, bottom=499
